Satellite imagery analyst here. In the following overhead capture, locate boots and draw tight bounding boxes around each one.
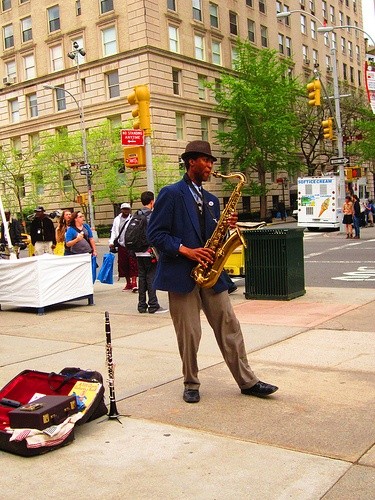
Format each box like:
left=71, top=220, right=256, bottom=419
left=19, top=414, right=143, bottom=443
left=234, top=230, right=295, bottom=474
left=346, top=233, right=353, bottom=239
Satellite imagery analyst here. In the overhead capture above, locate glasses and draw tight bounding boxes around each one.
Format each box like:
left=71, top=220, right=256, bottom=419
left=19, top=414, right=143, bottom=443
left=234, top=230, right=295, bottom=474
left=76, top=215, right=85, bottom=218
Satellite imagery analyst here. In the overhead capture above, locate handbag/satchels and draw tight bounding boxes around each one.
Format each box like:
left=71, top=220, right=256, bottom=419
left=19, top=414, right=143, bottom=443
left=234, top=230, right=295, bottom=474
left=47, top=367, right=108, bottom=424
left=96, top=253, right=115, bottom=284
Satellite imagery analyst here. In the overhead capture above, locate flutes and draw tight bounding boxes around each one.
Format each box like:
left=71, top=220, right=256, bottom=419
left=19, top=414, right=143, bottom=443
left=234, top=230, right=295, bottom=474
left=104, top=311, right=120, bottom=418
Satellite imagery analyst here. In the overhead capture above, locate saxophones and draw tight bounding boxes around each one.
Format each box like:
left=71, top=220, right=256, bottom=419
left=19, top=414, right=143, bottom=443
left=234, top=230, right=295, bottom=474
left=189, top=170, right=249, bottom=289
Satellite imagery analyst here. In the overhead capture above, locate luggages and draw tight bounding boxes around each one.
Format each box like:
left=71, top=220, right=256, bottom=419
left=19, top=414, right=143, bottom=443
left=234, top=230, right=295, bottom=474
left=0, top=369, right=105, bottom=458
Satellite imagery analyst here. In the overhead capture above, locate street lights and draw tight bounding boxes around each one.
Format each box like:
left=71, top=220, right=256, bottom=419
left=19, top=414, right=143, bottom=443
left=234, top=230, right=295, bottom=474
left=274, top=9, right=347, bottom=233
left=43, top=84, right=98, bottom=243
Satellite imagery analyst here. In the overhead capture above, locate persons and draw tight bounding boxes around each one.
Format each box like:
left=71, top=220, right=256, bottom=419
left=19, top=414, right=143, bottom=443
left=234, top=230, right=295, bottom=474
left=351, top=195, right=361, bottom=240
left=135, top=191, right=169, bottom=314
left=64, top=211, right=96, bottom=258
left=143, top=140, right=280, bottom=404
left=29, top=206, right=56, bottom=255
left=357, top=199, right=375, bottom=228
left=53, top=208, right=73, bottom=254
left=342, top=196, right=355, bottom=239
left=1, top=208, right=21, bottom=259
left=107, top=202, right=140, bottom=293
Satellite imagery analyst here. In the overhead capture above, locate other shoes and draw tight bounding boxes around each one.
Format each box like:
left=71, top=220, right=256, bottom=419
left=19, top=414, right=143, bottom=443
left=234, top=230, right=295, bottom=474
left=353, top=235, right=360, bottom=239
left=122, top=283, right=132, bottom=292
left=149, top=305, right=170, bottom=313
left=131, top=282, right=139, bottom=292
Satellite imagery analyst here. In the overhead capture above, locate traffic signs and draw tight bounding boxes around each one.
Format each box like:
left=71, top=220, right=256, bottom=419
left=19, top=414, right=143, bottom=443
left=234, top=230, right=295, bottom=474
left=80, top=170, right=92, bottom=175
left=121, top=129, right=144, bottom=146
left=329, top=156, right=350, bottom=165
left=80, top=164, right=91, bottom=170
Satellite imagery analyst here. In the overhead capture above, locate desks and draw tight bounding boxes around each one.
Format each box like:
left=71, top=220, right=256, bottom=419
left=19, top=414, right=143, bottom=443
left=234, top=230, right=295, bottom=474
left=0, top=252, right=94, bottom=316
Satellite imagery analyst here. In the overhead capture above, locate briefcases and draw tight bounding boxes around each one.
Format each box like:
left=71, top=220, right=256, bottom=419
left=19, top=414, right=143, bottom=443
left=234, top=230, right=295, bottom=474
left=8, top=395, right=78, bottom=431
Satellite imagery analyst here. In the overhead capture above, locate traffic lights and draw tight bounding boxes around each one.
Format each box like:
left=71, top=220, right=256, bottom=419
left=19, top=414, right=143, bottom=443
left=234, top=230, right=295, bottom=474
left=126, top=86, right=143, bottom=129
left=321, top=117, right=333, bottom=140
left=306, top=80, right=320, bottom=106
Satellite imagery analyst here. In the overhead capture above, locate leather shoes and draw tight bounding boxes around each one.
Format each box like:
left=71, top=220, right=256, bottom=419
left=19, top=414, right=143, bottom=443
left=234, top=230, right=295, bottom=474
left=241, top=380, right=279, bottom=396
left=183, top=388, right=201, bottom=402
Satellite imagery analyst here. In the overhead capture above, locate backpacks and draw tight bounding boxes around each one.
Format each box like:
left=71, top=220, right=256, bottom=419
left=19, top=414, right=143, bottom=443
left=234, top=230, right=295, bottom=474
left=124, top=209, right=154, bottom=252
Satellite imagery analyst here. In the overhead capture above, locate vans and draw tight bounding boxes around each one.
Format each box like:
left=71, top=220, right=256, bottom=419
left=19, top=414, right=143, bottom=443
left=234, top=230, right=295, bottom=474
left=296, top=174, right=368, bottom=231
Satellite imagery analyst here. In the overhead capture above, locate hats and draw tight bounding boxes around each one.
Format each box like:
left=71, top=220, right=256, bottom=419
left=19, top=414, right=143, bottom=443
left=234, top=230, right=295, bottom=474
left=34, top=206, right=46, bottom=211
left=181, top=140, right=218, bottom=161
left=120, top=203, right=131, bottom=209
